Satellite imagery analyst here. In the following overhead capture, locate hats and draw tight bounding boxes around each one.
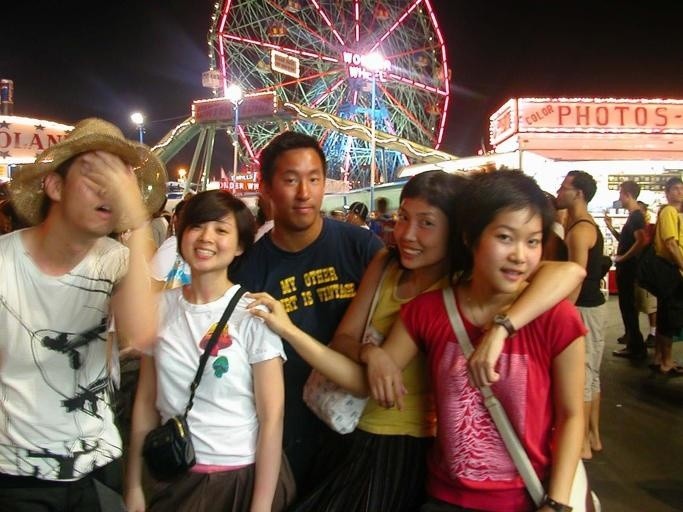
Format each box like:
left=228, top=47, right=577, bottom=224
left=9, top=118, right=166, bottom=232
left=331, top=210, right=344, bottom=215
left=343, top=202, right=368, bottom=218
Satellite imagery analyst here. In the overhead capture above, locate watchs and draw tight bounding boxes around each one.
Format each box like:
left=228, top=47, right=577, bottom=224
left=490, top=310, right=518, bottom=337
left=542, top=495, right=573, bottom=510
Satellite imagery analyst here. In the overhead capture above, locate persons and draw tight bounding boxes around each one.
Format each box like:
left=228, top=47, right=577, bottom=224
left=347, top=201, right=371, bottom=232
left=331, top=207, right=346, bottom=224
left=604, top=182, right=649, bottom=357
left=652, top=178, right=683, bottom=377
left=553, top=170, right=603, bottom=460
left=147, top=204, right=192, bottom=300
left=0, top=119, right=167, bottom=512
left=232, top=132, right=387, bottom=512
left=122, top=190, right=286, bottom=512
left=373, top=197, right=398, bottom=247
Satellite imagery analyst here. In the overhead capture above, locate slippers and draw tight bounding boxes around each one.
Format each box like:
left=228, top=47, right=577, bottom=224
left=591, top=441, right=605, bottom=456
left=651, top=364, right=661, bottom=369
left=660, top=366, right=683, bottom=377
left=582, top=457, right=594, bottom=465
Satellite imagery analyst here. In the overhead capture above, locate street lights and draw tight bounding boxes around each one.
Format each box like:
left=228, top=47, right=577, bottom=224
left=223, top=83, right=242, bottom=186
left=362, top=51, right=386, bottom=218
left=129, top=112, right=145, bottom=147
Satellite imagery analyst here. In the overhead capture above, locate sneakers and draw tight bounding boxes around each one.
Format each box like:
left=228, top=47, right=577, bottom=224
left=617, top=333, right=643, bottom=344
left=613, top=345, right=648, bottom=358
left=645, top=334, right=657, bottom=348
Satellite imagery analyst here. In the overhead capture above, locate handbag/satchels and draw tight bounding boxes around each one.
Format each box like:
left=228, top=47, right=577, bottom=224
left=303, top=258, right=388, bottom=435
left=143, top=286, right=248, bottom=481
left=442, top=286, right=600, bottom=512
left=639, top=205, right=680, bottom=299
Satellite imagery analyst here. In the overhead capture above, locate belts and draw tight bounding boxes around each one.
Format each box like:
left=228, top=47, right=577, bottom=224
left=1, top=463, right=106, bottom=489
left=615, top=262, right=635, bottom=267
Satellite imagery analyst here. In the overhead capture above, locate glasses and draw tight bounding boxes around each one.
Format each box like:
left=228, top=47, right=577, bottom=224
left=561, top=185, right=578, bottom=192
